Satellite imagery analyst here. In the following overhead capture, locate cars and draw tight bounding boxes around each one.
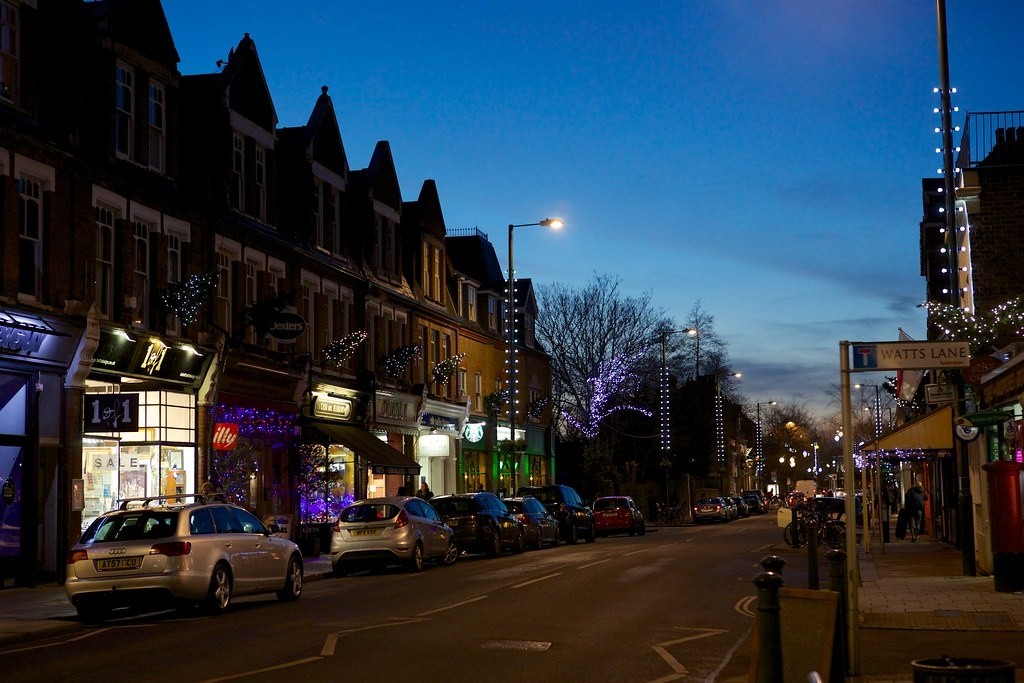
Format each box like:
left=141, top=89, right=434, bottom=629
left=430, top=493, right=526, bottom=559
left=692, top=497, right=728, bottom=524
left=592, top=496, right=646, bottom=537
left=518, top=485, right=597, bottom=545
left=330, top=497, right=458, bottom=576
left=723, top=497, right=738, bottom=520
left=732, top=489, right=844, bottom=518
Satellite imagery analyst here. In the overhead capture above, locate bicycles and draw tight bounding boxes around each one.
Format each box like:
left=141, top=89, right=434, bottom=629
left=653, top=502, right=685, bottom=527
left=784, top=505, right=846, bottom=552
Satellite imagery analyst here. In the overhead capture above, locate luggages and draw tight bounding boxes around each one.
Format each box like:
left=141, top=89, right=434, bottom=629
left=895, top=508, right=909, bottom=539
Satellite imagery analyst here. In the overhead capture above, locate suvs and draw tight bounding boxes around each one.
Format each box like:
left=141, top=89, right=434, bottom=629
left=65, top=495, right=304, bottom=621
left=500, top=495, right=561, bottom=550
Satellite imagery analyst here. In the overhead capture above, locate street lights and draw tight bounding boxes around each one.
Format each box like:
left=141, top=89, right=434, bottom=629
left=813, top=442, right=820, bottom=474
left=508, top=219, right=562, bottom=496
left=854, top=384, right=884, bottom=554
left=757, top=401, right=772, bottom=491
left=663, top=328, right=697, bottom=499
left=716, top=373, right=741, bottom=496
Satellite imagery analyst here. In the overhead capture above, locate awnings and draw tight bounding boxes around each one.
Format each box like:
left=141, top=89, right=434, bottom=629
left=307, top=422, right=420, bottom=474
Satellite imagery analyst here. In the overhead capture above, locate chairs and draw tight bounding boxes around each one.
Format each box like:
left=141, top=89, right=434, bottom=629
left=118, top=525, right=136, bottom=539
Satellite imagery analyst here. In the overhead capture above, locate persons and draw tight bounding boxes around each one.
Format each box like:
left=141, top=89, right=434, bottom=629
left=476, top=483, right=507, bottom=498
left=396, top=482, right=436, bottom=501
left=904, top=482, right=929, bottom=542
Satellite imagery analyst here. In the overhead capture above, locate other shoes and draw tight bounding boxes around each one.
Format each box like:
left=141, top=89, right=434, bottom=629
left=912, top=537, right=918, bottom=542
